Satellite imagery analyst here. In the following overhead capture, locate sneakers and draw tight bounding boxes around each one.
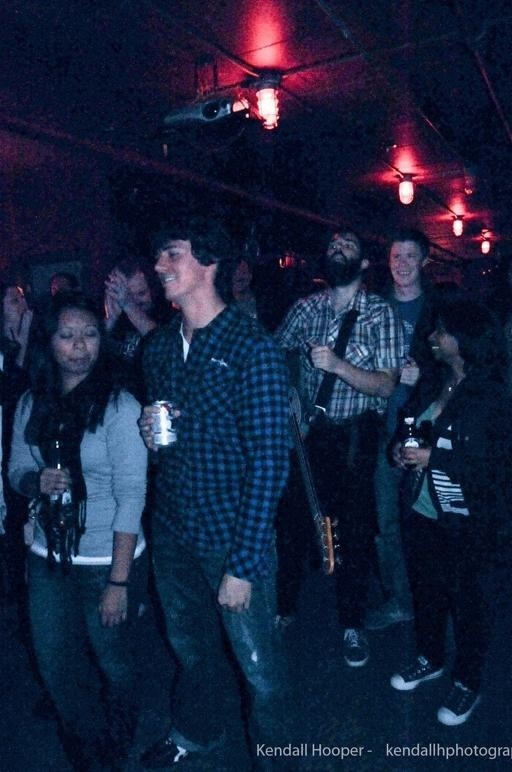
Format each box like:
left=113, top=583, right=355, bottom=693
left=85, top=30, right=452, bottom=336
left=364, top=597, right=416, bottom=631
left=437, top=682, right=481, bottom=727
left=389, top=653, right=444, bottom=691
left=343, top=627, right=371, bottom=667
left=140, top=736, right=195, bottom=769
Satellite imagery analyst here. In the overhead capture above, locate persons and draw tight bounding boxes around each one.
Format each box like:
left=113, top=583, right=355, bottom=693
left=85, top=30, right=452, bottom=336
left=0, top=222, right=511, bottom=771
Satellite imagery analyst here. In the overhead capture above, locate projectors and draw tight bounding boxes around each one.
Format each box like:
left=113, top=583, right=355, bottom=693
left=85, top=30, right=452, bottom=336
left=160, top=90, right=264, bottom=150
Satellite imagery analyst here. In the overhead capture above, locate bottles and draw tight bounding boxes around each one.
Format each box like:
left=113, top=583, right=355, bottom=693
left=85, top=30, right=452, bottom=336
left=42, top=436, right=78, bottom=533
left=399, top=414, right=423, bottom=471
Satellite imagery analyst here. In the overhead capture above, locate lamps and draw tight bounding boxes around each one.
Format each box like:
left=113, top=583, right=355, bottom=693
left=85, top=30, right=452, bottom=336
left=398, top=171, right=490, bottom=254
left=245, top=67, right=283, bottom=130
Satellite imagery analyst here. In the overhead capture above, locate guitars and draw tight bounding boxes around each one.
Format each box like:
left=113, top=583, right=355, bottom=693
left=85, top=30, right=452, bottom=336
left=285, top=347, right=342, bottom=578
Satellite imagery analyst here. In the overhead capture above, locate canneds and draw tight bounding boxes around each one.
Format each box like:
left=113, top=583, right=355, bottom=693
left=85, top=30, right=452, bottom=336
left=150, top=400, right=178, bottom=448
